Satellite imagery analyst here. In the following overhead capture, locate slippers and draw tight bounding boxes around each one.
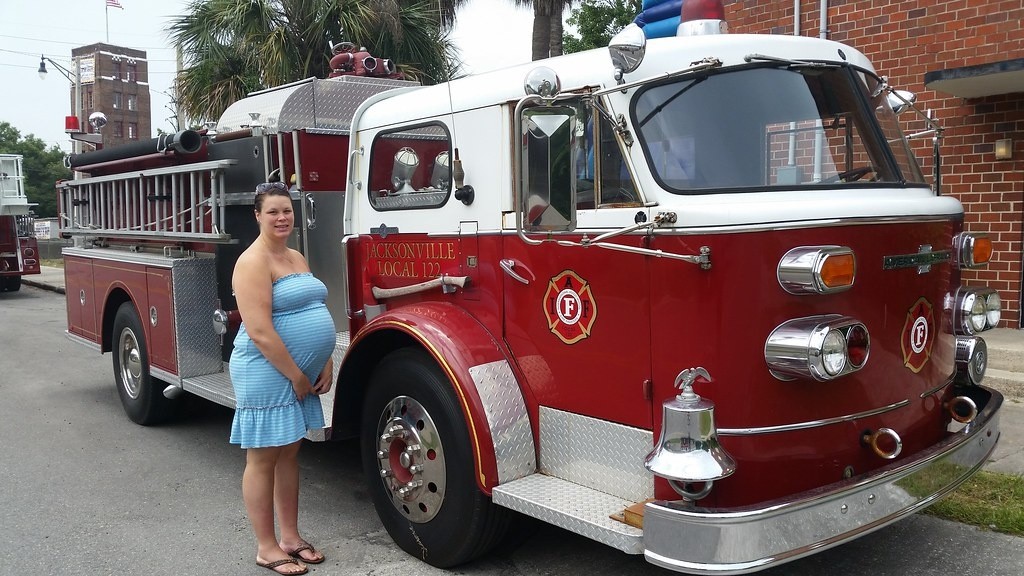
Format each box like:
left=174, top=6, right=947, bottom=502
left=289, top=544, right=325, bottom=563
left=256, top=555, right=309, bottom=576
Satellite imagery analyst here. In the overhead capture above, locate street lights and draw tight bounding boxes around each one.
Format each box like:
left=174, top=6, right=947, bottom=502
left=38, top=55, right=83, bottom=239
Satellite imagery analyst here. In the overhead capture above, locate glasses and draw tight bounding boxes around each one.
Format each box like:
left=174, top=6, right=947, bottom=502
left=255, top=182, right=289, bottom=196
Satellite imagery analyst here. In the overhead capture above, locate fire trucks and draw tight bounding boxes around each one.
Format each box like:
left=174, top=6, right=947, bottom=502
left=0, top=155, right=40, bottom=292
left=57, top=0, right=1002, bottom=576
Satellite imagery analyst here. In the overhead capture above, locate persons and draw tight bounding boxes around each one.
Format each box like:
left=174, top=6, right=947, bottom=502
left=230, top=182, right=337, bottom=576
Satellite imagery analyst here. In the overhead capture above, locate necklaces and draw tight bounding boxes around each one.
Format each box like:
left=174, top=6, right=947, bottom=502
left=262, top=234, right=293, bottom=265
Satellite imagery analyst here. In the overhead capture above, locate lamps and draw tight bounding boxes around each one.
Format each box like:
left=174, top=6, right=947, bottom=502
left=994, top=139, right=1012, bottom=159
